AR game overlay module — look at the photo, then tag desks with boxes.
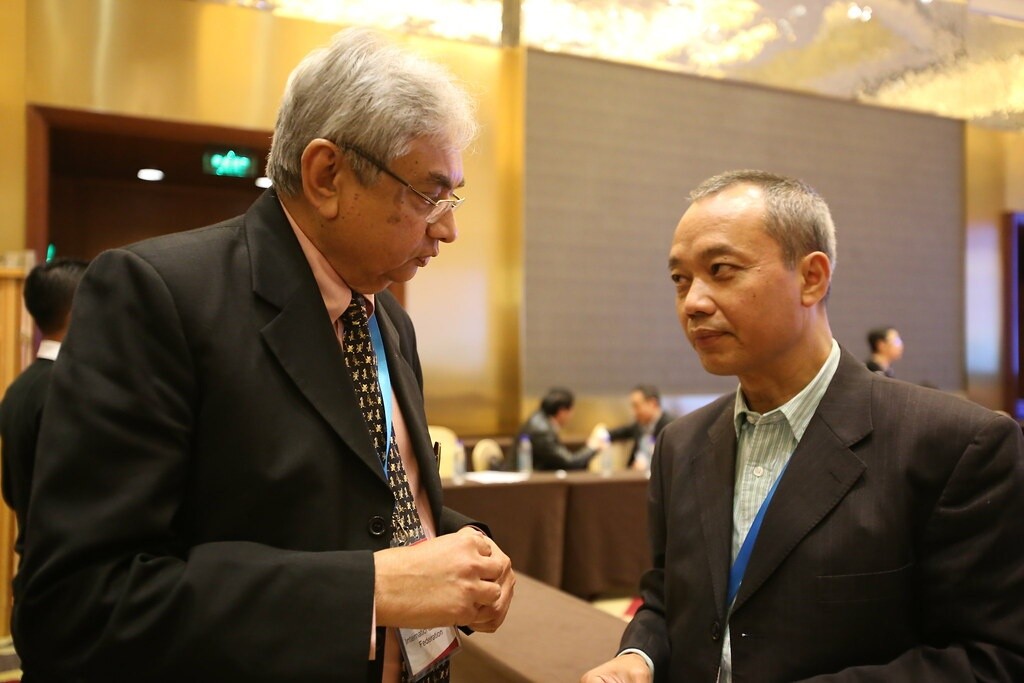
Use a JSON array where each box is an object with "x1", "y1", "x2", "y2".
[{"x1": 439, "y1": 467, "x2": 652, "y2": 683}]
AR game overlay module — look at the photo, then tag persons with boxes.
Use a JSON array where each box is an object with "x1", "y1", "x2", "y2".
[
  {"x1": 0, "y1": 259, "x2": 88, "y2": 683},
  {"x1": 495, "y1": 381, "x2": 675, "y2": 472},
  {"x1": 864, "y1": 325, "x2": 904, "y2": 378},
  {"x1": 7, "y1": 25, "x2": 514, "y2": 683},
  {"x1": 578, "y1": 168, "x2": 1024, "y2": 683}
]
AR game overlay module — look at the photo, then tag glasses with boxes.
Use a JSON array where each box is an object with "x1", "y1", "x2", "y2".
[{"x1": 330, "y1": 140, "x2": 466, "y2": 224}]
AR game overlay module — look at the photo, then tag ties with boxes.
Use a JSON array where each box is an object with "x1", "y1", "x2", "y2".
[{"x1": 335, "y1": 292, "x2": 449, "y2": 683}]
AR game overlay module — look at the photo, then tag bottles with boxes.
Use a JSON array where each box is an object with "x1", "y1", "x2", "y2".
[{"x1": 517, "y1": 433, "x2": 533, "y2": 472}]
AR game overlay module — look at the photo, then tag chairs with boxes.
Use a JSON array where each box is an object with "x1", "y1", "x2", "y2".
[
  {"x1": 588, "y1": 419, "x2": 636, "y2": 471},
  {"x1": 429, "y1": 426, "x2": 458, "y2": 473},
  {"x1": 471, "y1": 439, "x2": 504, "y2": 471}
]
